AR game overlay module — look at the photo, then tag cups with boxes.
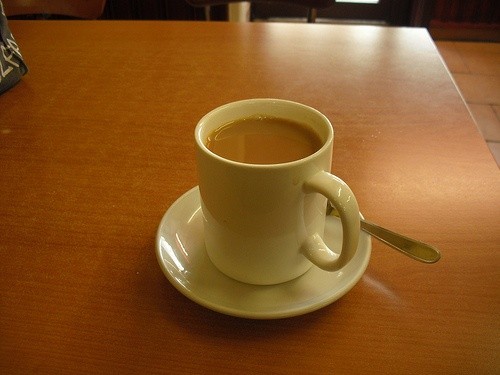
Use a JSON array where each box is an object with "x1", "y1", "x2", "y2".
[{"x1": 194, "y1": 98, "x2": 360, "y2": 286}]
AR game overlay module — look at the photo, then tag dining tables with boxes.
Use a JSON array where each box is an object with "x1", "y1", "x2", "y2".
[{"x1": 0, "y1": 18, "x2": 499, "y2": 375}]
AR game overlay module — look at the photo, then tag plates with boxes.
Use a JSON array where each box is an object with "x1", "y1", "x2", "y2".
[{"x1": 154, "y1": 184, "x2": 371, "y2": 320}]
